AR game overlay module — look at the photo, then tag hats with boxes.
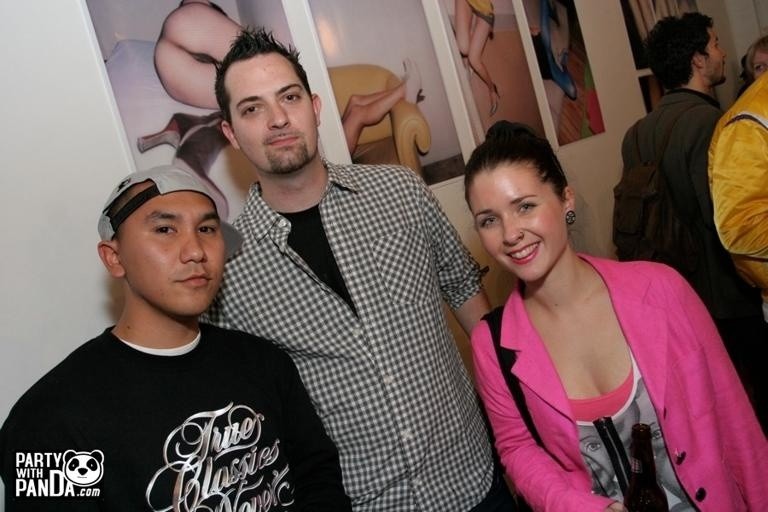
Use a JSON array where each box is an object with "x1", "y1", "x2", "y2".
[{"x1": 97, "y1": 167, "x2": 244, "y2": 262}]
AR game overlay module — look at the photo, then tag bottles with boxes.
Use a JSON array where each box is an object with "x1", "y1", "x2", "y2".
[{"x1": 623, "y1": 422, "x2": 669, "y2": 512}]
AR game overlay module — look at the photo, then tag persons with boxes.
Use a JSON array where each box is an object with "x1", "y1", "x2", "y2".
[
  {"x1": 136, "y1": 1, "x2": 247, "y2": 223},
  {"x1": 1, "y1": 164, "x2": 353, "y2": 512},
  {"x1": 468, "y1": 118, "x2": 767, "y2": 512},
  {"x1": 449, "y1": 0, "x2": 504, "y2": 117},
  {"x1": 191, "y1": 22, "x2": 519, "y2": 512},
  {"x1": 340, "y1": 57, "x2": 422, "y2": 155}
]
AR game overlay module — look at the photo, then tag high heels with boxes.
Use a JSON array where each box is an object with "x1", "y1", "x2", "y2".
[
  {"x1": 490, "y1": 83, "x2": 500, "y2": 116},
  {"x1": 403, "y1": 58, "x2": 426, "y2": 105},
  {"x1": 136, "y1": 112, "x2": 230, "y2": 214}
]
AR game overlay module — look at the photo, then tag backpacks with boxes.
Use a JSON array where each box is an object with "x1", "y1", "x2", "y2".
[{"x1": 612, "y1": 101, "x2": 706, "y2": 274}]
